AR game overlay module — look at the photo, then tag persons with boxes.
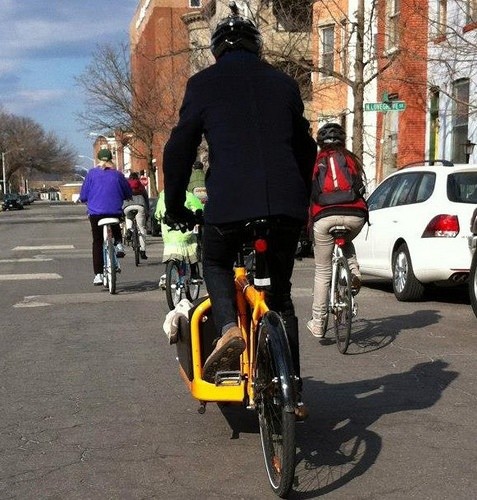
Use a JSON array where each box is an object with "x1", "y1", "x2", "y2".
[
  {"x1": 80, "y1": 149, "x2": 133, "y2": 285},
  {"x1": 307, "y1": 123, "x2": 369, "y2": 338},
  {"x1": 161, "y1": 16, "x2": 318, "y2": 420},
  {"x1": 186, "y1": 161, "x2": 208, "y2": 205},
  {"x1": 154, "y1": 186, "x2": 205, "y2": 288},
  {"x1": 122, "y1": 171, "x2": 150, "y2": 260},
  {"x1": 139, "y1": 170, "x2": 158, "y2": 217}
]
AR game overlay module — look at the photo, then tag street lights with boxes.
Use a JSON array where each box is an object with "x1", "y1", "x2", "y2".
[
  {"x1": 90, "y1": 132, "x2": 119, "y2": 171},
  {"x1": 2, "y1": 148, "x2": 24, "y2": 194},
  {"x1": 74, "y1": 155, "x2": 96, "y2": 181}
]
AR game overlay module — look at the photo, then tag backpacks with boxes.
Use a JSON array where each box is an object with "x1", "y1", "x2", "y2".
[
  {"x1": 312, "y1": 150, "x2": 363, "y2": 207},
  {"x1": 128, "y1": 178, "x2": 141, "y2": 192}
]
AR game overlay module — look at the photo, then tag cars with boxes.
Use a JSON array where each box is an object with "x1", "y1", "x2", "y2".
[
  {"x1": 146, "y1": 197, "x2": 160, "y2": 236},
  {"x1": 23, "y1": 194, "x2": 34, "y2": 205}
]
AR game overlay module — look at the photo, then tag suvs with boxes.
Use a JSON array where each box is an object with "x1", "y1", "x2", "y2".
[
  {"x1": 2, "y1": 193, "x2": 24, "y2": 211},
  {"x1": 336, "y1": 158, "x2": 477, "y2": 317}
]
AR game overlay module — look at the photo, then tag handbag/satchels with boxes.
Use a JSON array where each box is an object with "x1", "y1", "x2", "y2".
[{"x1": 176, "y1": 296, "x2": 218, "y2": 383}]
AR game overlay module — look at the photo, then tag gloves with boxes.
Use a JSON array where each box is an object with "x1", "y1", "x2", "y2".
[{"x1": 163, "y1": 205, "x2": 194, "y2": 233}]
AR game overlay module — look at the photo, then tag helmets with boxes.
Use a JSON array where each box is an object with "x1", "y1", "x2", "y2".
[
  {"x1": 316, "y1": 122, "x2": 345, "y2": 146},
  {"x1": 209, "y1": 16, "x2": 262, "y2": 57}
]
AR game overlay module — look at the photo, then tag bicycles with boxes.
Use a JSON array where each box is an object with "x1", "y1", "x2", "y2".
[
  {"x1": 124, "y1": 209, "x2": 149, "y2": 266},
  {"x1": 161, "y1": 209, "x2": 308, "y2": 498},
  {"x1": 78, "y1": 198, "x2": 132, "y2": 295},
  {"x1": 161, "y1": 246, "x2": 203, "y2": 311},
  {"x1": 312, "y1": 225, "x2": 360, "y2": 355}
]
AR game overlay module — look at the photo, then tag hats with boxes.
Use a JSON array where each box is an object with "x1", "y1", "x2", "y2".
[{"x1": 98, "y1": 149, "x2": 112, "y2": 162}]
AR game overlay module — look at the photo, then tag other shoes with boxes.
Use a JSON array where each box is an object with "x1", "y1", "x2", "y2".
[
  {"x1": 293, "y1": 405, "x2": 307, "y2": 421},
  {"x1": 203, "y1": 327, "x2": 247, "y2": 383},
  {"x1": 115, "y1": 247, "x2": 125, "y2": 257},
  {"x1": 351, "y1": 275, "x2": 360, "y2": 296},
  {"x1": 159, "y1": 274, "x2": 166, "y2": 287},
  {"x1": 191, "y1": 276, "x2": 203, "y2": 285},
  {"x1": 126, "y1": 229, "x2": 132, "y2": 240},
  {"x1": 306, "y1": 320, "x2": 323, "y2": 338},
  {"x1": 141, "y1": 251, "x2": 148, "y2": 259},
  {"x1": 93, "y1": 276, "x2": 102, "y2": 285}
]
